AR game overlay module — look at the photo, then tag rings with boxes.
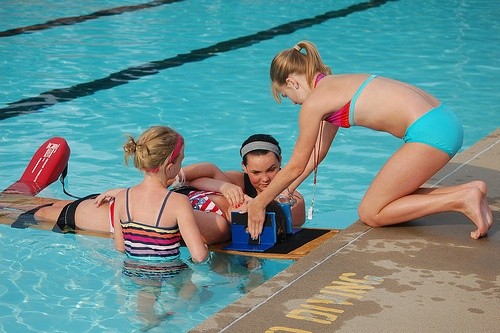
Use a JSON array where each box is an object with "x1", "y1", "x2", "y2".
[{"x1": 225, "y1": 195, "x2": 230, "y2": 197}]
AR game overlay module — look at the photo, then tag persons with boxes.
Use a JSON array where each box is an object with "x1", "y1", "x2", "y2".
[
  {"x1": 182, "y1": 134, "x2": 306, "y2": 293},
  {"x1": 1, "y1": 163, "x2": 287, "y2": 245},
  {"x1": 241, "y1": 41, "x2": 494, "y2": 240},
  {"x1": 113, "y1": 125, "x2": 208, "y2": 332}
]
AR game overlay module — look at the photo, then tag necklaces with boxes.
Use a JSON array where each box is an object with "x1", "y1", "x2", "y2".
[{"x1": 307, "y1": 73, "x2": 329, "y2": 219}]
